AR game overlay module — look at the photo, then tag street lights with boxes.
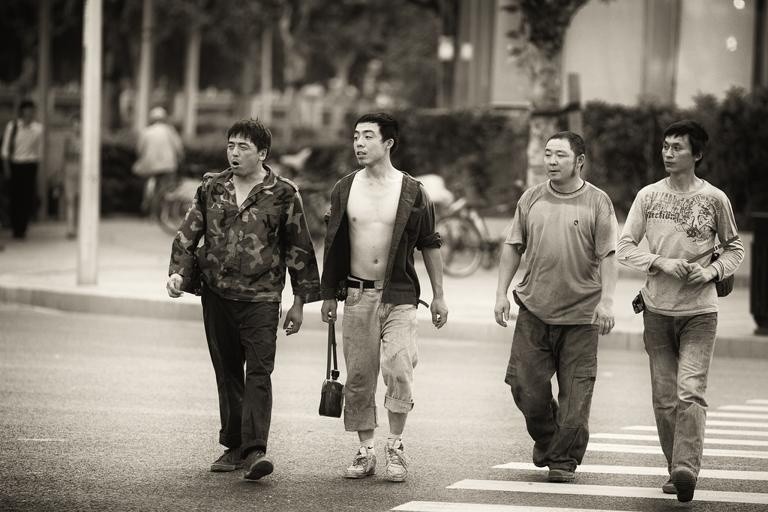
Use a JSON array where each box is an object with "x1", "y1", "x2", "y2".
[{"x1": 436, "y1": 32, "x2": 457, "y2": 111}]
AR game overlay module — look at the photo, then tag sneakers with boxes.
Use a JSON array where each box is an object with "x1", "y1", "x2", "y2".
[
  {"x1": 548, "y1": 469, "x2": 575, "y2": 482},
  {"x1": 532, "y1": 442, "x2": 546, "y2": 467},
  {"x1": 384, "y1": 439, "x2": 408, "y2": 481},
  {"x1": 662, "y1": 478, "x2": 676, "y2": 494},
  {"x1": 243, "y1": 450, "x2": 274, "y2": 480},
  {"x1": 343, "y1": 446, "x2": 377, "y2": 479},
  {"x1": 671, "y1": 467, "x2": 696, "y2": 502},
  {"x1": 210, "y1": 448, "x2": 242, "y2": 472}
]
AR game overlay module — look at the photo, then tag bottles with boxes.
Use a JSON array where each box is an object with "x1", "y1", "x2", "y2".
[{"x1": 319, "y1": 368, "x2": 345, "y2": 418}]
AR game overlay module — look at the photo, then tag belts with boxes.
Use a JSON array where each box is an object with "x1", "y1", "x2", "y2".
[{"x1": 345, "y1": 281, "x2": 373, "y2": 288}]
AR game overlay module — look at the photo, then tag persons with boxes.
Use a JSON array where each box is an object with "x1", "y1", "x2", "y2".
[
  {"x1": 55, "y1": 114, "x2": 80, "y2": 238},
  {"x1": 132, "y1": 107, "x2": 186, "y2": 216},
  {"x1": 321, "y1": 113, "x2": 448, "y2": 482},
  {"x1": 495, "y1": 131, "x2": 621, "y2": 482},
  {"x1": 1, "y1": 98, "x2": 44, "y2": 239},
  {"x1": 616, "y1": 119, "x2": 745, "y2": 502},
  {"x1": 166, "y1": 117, "x2": 321, "y2": 480}
]
